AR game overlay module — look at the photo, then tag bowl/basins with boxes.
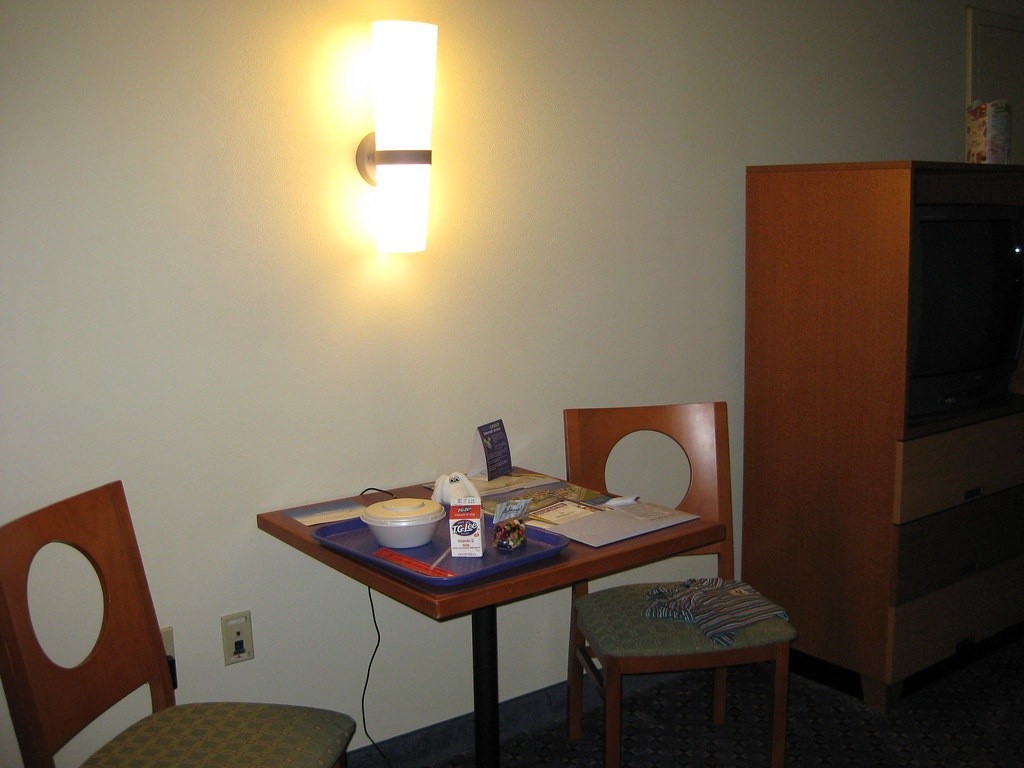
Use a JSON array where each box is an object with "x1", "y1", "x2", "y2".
[{"x1": 360, "y1": 498, "x2": 446, "y2": 549}]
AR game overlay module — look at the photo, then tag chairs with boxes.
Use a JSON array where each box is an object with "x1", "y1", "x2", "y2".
[
  {"x1": 0, "y1": 481, "x2": 356, "y2": 768},
  {"x1": 563, "y1": 402, "x2": 797, "y2": 768}
]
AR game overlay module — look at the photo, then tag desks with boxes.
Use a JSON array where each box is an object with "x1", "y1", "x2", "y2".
[{"x1": 257, "y1": 466, "x2": 726, "y2": 768}]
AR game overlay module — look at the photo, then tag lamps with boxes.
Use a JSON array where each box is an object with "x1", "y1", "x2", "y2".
[{"x1": 356, "y1": 21, "x2": 438, "y2": 252}]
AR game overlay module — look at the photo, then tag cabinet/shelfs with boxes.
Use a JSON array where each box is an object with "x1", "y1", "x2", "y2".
[{"x1": 740, "y1": 160, "x2": 1024, "y2": 712}]
